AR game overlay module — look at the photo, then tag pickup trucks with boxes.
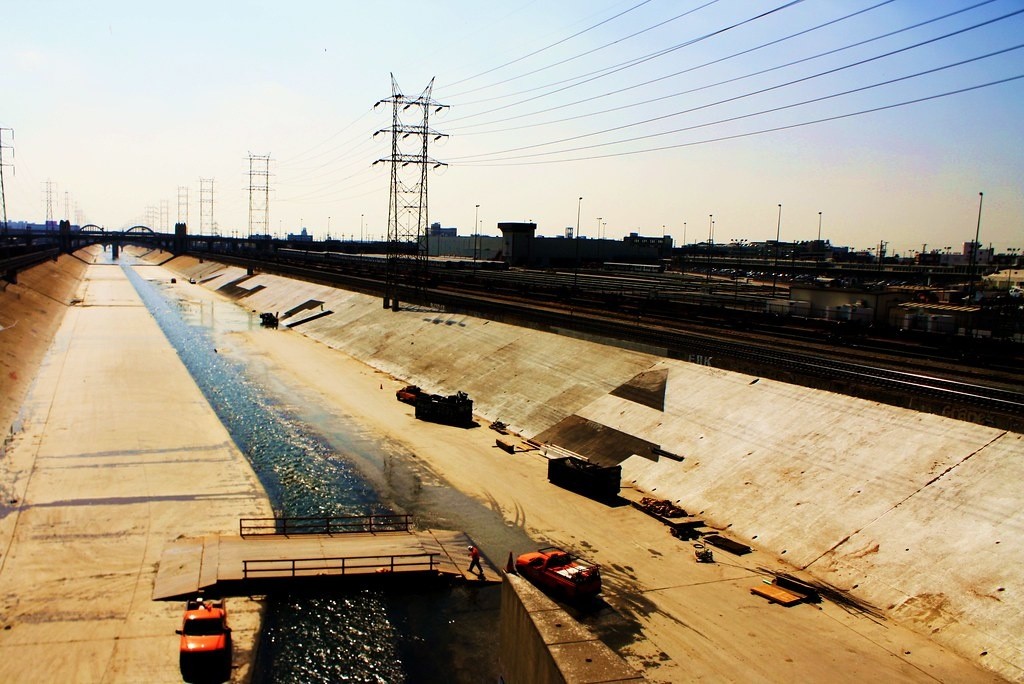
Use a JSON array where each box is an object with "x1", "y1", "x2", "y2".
[
  {"x1": 516, "y1": 550, "x2": 605, "y2": 607},
  {"x1": 175, "y1": 599, "x2": 233, "y2": 666}
]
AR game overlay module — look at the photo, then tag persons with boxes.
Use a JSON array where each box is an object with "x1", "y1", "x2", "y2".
[{"x1": 467, "y1": 546, "x2": 484, "y2": 576}]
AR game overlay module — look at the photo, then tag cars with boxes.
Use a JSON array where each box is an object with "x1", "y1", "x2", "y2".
[{"x1": 396, "y1": 384, "x2": 426, "y2": 403}]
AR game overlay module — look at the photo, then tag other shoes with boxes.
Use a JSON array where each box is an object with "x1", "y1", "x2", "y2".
[
  {"x1": 467, "y1": 569, "x2": 472, "y2": 572},
  {"x1": 480, "y1": 570, "x2": 483, "y2": 573}
]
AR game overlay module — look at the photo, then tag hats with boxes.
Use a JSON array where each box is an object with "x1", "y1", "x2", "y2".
[{"x1": 468, "y1": 546, "x2": 473, "y2": 550}]
